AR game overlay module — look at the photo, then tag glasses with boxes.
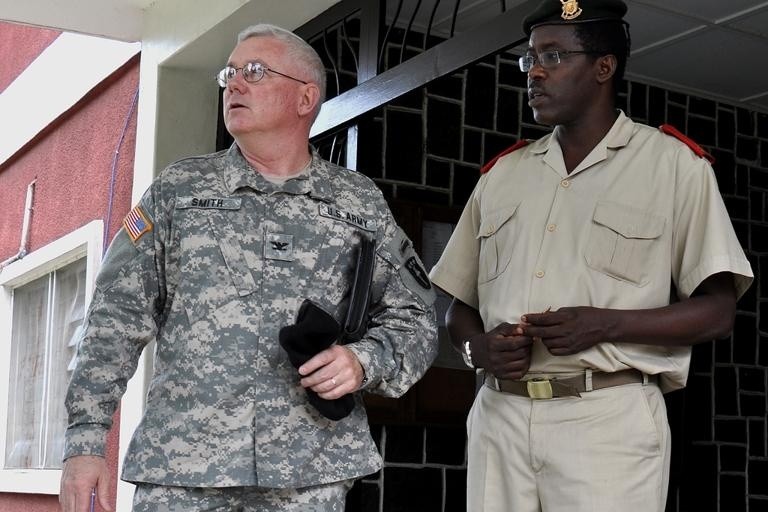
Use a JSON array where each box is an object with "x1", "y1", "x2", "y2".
[
  {"x1": 214, "y1": 62, "x2": 308, "y2": 88},
  {"x1": 518, "y1": 50, "x2": 586, "y2": 73}
]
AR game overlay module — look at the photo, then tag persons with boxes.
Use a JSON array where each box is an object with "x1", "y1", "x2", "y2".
[
  {"x1": 423, "y1": 0, "x2": 757, "y2": 511},
  {"x1": 55, "y1": 20, "x2": 441, "y2": 511}
]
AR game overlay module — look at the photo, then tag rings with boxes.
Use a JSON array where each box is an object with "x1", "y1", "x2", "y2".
[{"x1": 330, "y1": 377, "x2": 337, "y2": 386}]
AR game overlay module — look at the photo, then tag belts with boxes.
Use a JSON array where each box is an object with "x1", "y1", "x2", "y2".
[{"x1": 483, "y1": 368, "x2": 655, "y2": 400}]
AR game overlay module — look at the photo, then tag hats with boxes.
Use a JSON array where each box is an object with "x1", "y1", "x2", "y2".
[
  {"x1": 278, "y1": 301, "x2": 356, "y2": 421},
  {"x1": 521, "y1": 0, "x2": 627, "y2": 36}
]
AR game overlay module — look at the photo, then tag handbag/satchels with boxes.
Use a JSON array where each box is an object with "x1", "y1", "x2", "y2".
[{"x1": 339, "y1": 238, "x2": 376, "y2": 346}]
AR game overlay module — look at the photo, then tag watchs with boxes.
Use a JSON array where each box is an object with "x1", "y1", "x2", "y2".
[{"x1": 460, "y1": 338, "x2": 476, "y2": 370}]
